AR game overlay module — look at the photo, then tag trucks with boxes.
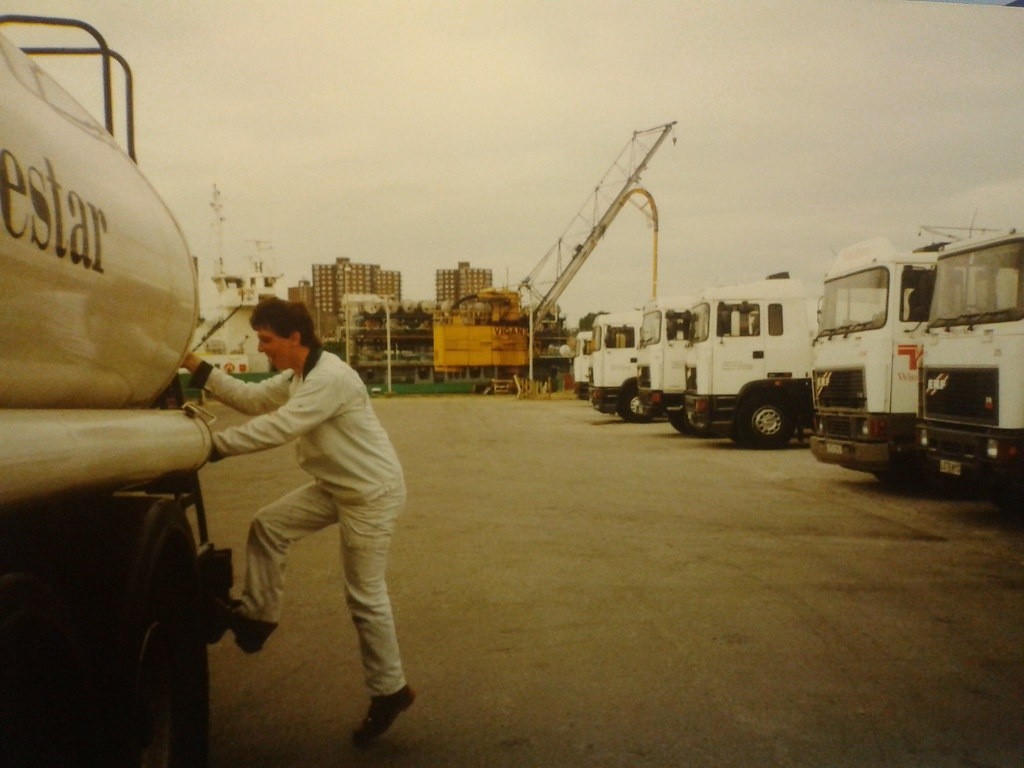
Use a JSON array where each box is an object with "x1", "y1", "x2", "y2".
[
  {"x1": 573, "y1": 332, "x2": 626, "y2": 400},
  {"x1": 810, "y1": 234, "x2": 1017, "y2": 499},
  {"x1": 587, "y1": 310, "x2": 683, "y2": 423},
  {"x1": 683, "y1": 271, "x2": 913, "y2": 451},
  {"x1": 919, "y1": 229, "x2": 1024, "y2": 521},
  {"x1": 0, "y1": 15, "x2": 233, "y2": 768},
  {"x1": 636, "y1": 299, "x2": 759, "y2": 437}
]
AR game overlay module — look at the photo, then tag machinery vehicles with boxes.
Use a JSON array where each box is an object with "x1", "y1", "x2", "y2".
[{"x1": 431, "y1": 121, "x2": 678, "y2": 374}]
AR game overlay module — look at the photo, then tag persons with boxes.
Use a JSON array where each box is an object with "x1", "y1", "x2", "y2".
[{"x1": 183, "y1": 295, "x2": 414, "y2": 747}]
